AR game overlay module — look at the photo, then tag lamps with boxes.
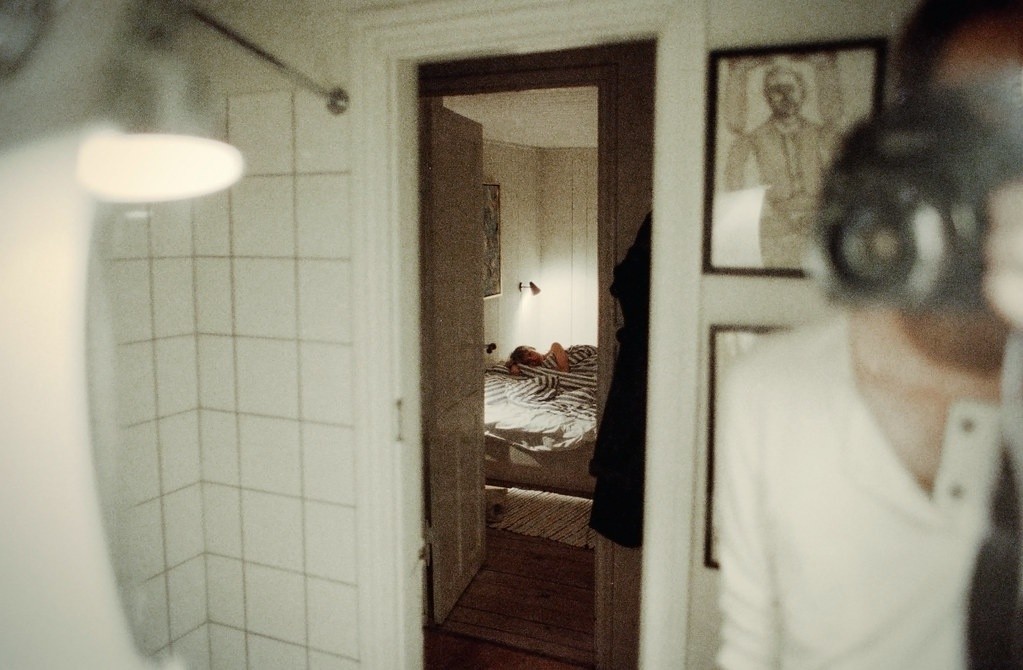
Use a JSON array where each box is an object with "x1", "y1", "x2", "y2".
[
  {"x1": 81, "y1": 1, "x2": 350, "y2": 206},
  {"x1": 519, "y1": 281, "x2": 541, "y2": 296}
]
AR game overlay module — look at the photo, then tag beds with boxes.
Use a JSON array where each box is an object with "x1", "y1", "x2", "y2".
[{"x1": 482, "y1": 360, "x2": 595, "y2": 549}]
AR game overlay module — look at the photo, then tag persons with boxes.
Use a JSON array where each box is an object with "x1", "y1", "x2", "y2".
[
  {"x1": 715, "y1": 0, "x2": 1023, "y2": 670},
  {"x1": 505, "y1": 342, "x2": 569, "y2": 375}
]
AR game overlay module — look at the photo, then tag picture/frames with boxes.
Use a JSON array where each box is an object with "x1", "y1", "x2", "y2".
[
  {"x1": 702, "y1": 36, "x2": 886, "y2": 279},
  {"x1": 705, "y1": 323, "x2": 789, "y2": 570},
  {"x1": 482, "y1": 183, "x2": 503, "y2": 300}
]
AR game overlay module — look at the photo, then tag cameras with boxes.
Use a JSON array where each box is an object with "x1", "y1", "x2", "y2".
[{"x1": 810, "y1": 83, "x2": 1023, "y2": 316}]
